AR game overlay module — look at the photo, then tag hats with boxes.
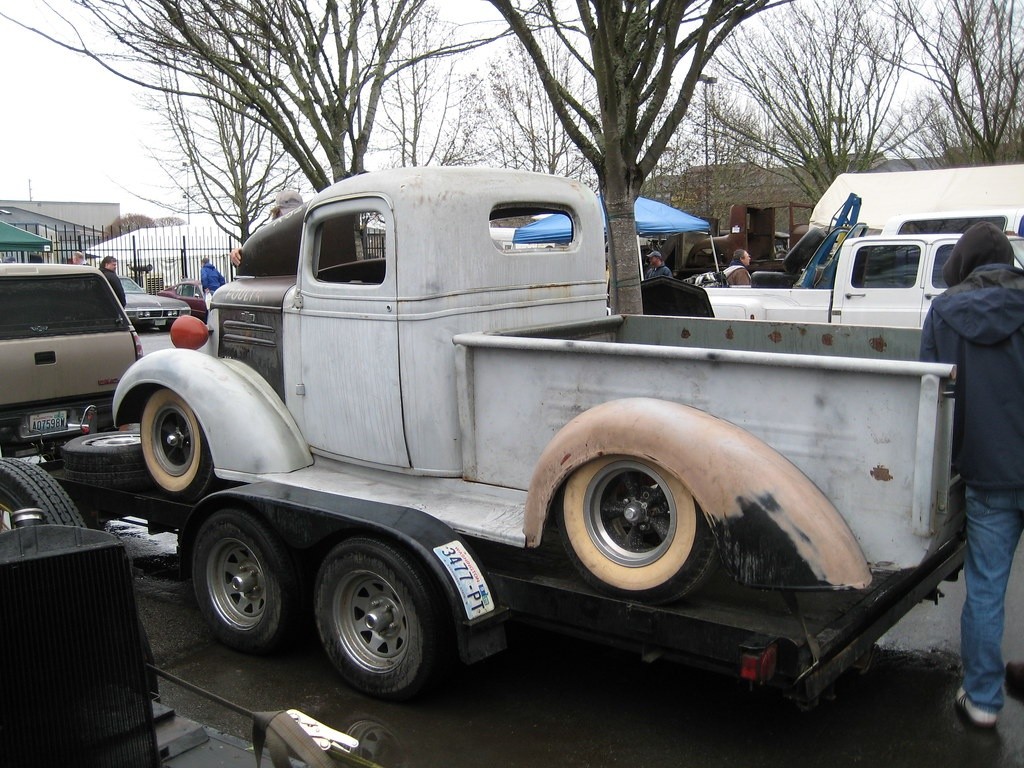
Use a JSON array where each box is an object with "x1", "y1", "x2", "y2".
[{"x1": 646, "y1": 252, "x2": 662, "y2": 258}]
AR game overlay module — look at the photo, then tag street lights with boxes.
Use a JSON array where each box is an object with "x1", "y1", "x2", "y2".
[{"x1": 697, "y1": 74, "x2": 717, "y2": 216}]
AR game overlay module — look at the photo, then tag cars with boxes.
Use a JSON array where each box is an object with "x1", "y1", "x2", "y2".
[
  {"x1": 116, "y1": 275, "x2": 191, "y2": 332},
  {"x1": 157, "y1": 279, "x2": 208, "y2": 324},
  {"x1": 0, "y1": 263, "x2": 143, "y2": 456}
]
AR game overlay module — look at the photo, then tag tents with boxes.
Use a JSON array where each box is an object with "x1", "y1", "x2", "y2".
[
  {"x1": 512, "y1": 195, "x2": 719, "y2": 282},
  {"x1": 0, "y1": 220, "x2": 53, "y2": 264}
]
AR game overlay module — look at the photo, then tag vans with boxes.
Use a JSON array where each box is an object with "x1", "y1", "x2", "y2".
[{"x1": 808, "y1": 163, "x2": 1023, "y2": 237}]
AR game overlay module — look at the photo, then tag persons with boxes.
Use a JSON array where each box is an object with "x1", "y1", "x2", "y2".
[
  {"x1": 98, "y1": 256, "x2": 128, "y2": 309},
  {"x1": 73, "y1": 252, "x2": 90, "y2": 266},
  {"x1": 645, "y1": 251, "x2": 672, "y2": 280},
  {"x1": 230, "y1": 190, "x2": 304, "y2": 268},
  {"x1": 723, "y1": 250, "x2": 751, "y2": 285},
  {"x1": 919, "y1": 222, "x2": 1024, "y2": 724},
  {"x1": 3, "y1": 253, "x2": 44, "y2": 263},
  {"x1": 201, "y1": 258, "x2": 225, "y2": 310}
]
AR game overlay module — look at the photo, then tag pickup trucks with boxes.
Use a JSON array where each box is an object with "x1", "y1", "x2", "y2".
[
  {"x1": 702, "y1": 233, "x2": 1024, "y2": 329},
  {"x1": 111, "y1": 167, "x2": 958, "y2": 609}
]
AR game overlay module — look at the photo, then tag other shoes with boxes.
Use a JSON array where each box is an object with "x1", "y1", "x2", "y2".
[{"x1": 957, "y1": 686, "x2": 997, "y2": 727}]
